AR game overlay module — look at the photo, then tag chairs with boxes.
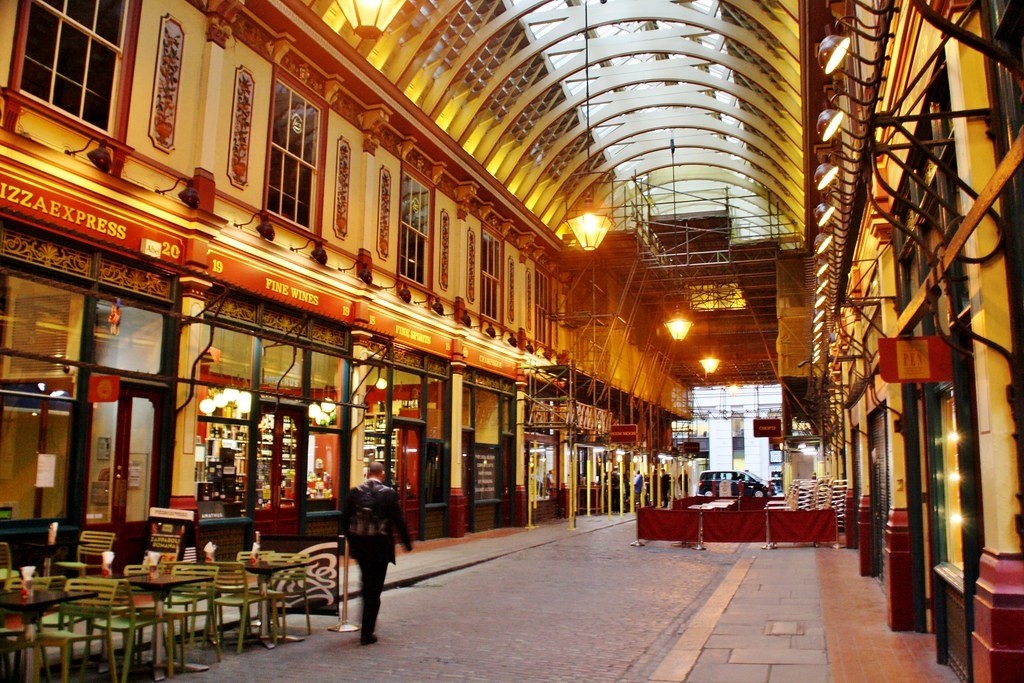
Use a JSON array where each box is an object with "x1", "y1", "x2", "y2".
[{"x1": 0, "y1": 531, "x2": 311, "y2": 683}]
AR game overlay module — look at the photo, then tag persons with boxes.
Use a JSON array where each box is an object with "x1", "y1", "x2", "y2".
[
  {"x1": 546, "y1": 470, "x2": 554, "y2": 488},
  {"x1": 343, "y1": 460, "x2": 413, "y2": 645},
  {"x1": 611, "y1": 465, "x2": 688, "y2": 513}
]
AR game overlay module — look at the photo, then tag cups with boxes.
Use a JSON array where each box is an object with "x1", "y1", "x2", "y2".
[
  {"x1": 250, "y1": 552, "x2": 259, "y2": 565},
  {"x1": 22, "y1": 580, "x2": 33, "y2": 598},
  {"x1": 205, "y1": 552, "x2": 215, "y2": 561},
  {"x1": 149, "y1": 565, "x2": 158, "y2": 578},
  {"x1": 102, "y1": 564, "x2": 112, "y2": 578}
]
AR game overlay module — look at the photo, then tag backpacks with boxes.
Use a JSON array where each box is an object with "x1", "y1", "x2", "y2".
[{"x1": 349, "y1": 485, "x2": 390, "y2": 535}]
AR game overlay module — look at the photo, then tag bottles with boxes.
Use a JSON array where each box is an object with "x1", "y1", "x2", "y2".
[{"x1": 364, "y1": 416, "x2": 395, "y2": 480}]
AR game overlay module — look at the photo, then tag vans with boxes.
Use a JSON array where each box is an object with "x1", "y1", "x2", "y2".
[{"x1": 698, "y1": 471, "x2": 777, "y2": 498}]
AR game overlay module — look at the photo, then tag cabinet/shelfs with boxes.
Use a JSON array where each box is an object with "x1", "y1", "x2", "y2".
[
  {"x1": 364, "y1": 413, "x2": 398, "y2": 479},
  {"x1": 205, "y1": 413, "x2": 296, "y2": 513}
]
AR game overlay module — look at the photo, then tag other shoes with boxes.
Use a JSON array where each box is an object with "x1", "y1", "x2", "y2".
[{"x1": 361, "y1": 635, "x2": 376, "y2": 645}]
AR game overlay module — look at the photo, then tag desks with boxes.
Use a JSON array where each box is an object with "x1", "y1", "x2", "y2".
[
  {"x1": 12, "y1": 538, "x2": 90, "y2": 577},
  {"x1": 111, "y1": 573, "x2": 214, "y2": 682},
  {"x1": 763, "y1": 501, "x2": 790, "y2": 511},
  {"x1": 223, "y1": 560, "x2": 312, "y2": 649},
  {"x1": 0, "y1": 588, "x2": 99, "y2": 683},
  {"x1": 688, "y1": 499, "x2": 736, "y2": 511}
]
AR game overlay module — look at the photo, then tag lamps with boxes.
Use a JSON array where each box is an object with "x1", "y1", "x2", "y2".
[
  {"x1": 664, "y1": 140, "x2": 694, "y2": 341},
  {"x1": 338, "y1": 260, "x2": 372, "y2": 286},
  {"x1": 335, "y1": 0, "x2": 406, "y2": 42},
  {"x1": 379, "y1": 280, "x2": 411, "y2": 303},
  {"x1": 234, "y1": 209, "x2": 275, "y2": 242},
  {"x1": 65, "y1": 137, "x2": 111, "y2": 173},
  {"x1": 818, "y1": 16, "x2": 880, "y2": 75},
  {"x1": 817, "y1": 93, "x2": 847, "y2": 142},
  {"x1": 810, "y1": 149, "x2": 857, "y2": 365},
  {"x1": 565, "y1": 0, "x2": 613, "y2": 250},
  {"x1": 698, "y1": 311, "x2": 721, "y2": 373},
  {"x1": 155, "y1": 178, "x2": 200, "y2": 210},
  {"x1": 290, "y1": 238, "x2": 328, "y2": 265},
  {"x1": 413, "y1": 294, "x2": 551, "y2": 362}
]
